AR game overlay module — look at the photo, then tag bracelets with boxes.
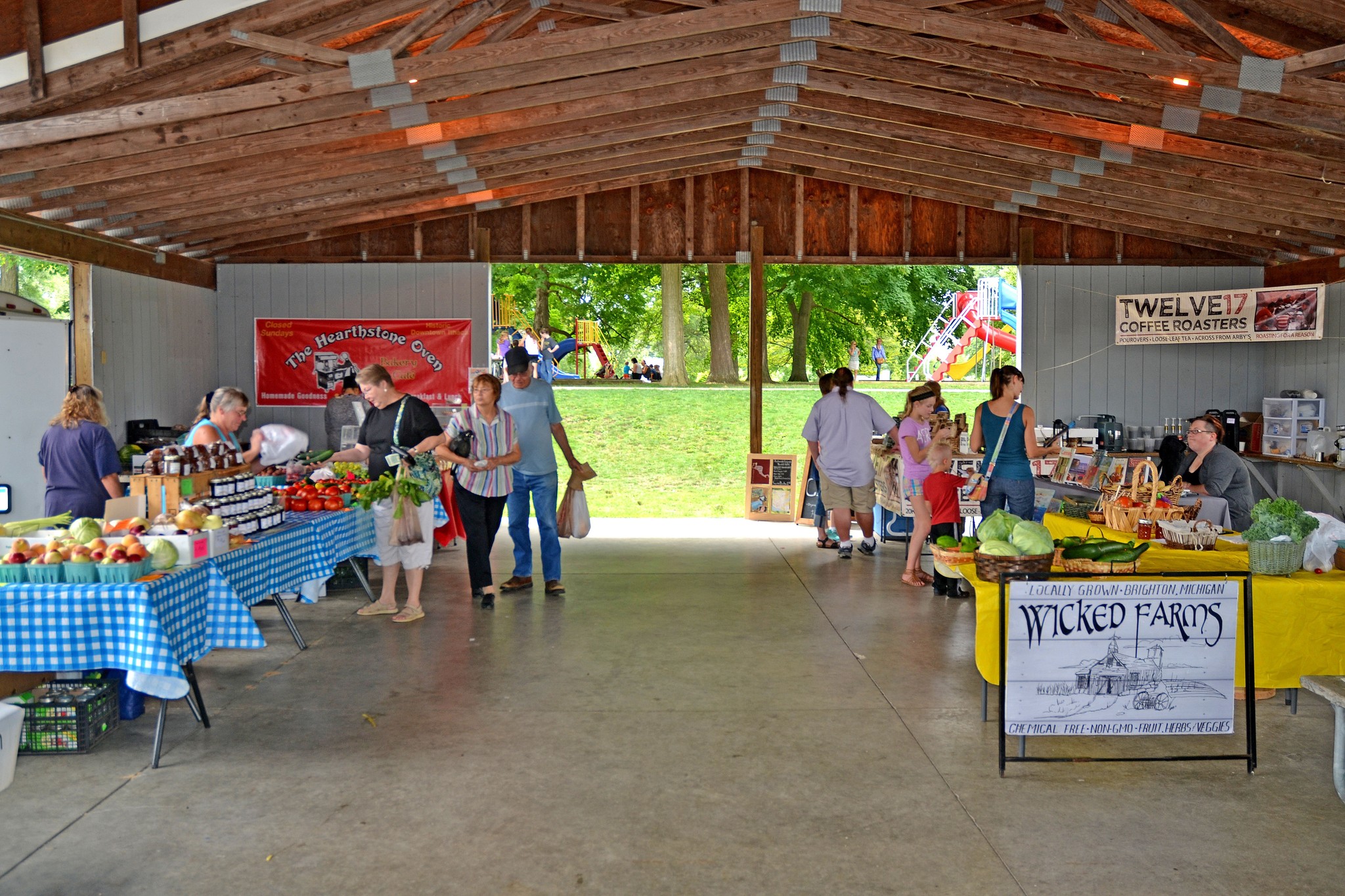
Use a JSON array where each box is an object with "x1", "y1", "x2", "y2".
[{"x1": 412, "y1": 447, "x2": 419, "y2": 454}]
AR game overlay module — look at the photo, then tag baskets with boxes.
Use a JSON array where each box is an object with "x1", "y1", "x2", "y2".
[
  {"x1": 12, "y1": 676, "x2": 122, "y2": 755},
  {"x1": 946, "y1": 423, "x2": 969, "y2": 452},
  {"x1": 929, "y1": 460, "x2": 1306, "y2": 584}
]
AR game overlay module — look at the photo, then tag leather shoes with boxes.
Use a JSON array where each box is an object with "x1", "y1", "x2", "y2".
[
  {"x1": 472, "y1": 586, "x2": 495, "y2": 610},
  {"x1": 500, "y1": 576, "x2": 533, "y2": 591},
  {"x1": 544, "y1": 580, "x2": 566, "y2": 596}
]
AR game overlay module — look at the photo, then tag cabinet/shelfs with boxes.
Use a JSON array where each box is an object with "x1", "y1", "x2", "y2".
[{"x1": 1261, "y1": 397, "x2": 1326, "y2": 459}]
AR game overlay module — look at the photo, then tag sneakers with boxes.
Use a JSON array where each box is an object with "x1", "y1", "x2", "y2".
[
  {"x1": 857, "y1": 536, "x2": 877, "y2": 556},
  {"x1": 838, "y1": 544, "x2": 853, "y2": 559}
]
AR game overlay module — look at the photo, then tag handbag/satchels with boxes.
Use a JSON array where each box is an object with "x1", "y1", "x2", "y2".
[
  {"x1": 449, "y1": 430, "x2": 475, "y2": 458},
  {"x1": 388, "y1": 462, "x2": 423, "y2": 545},
  {"x1": 397, "y1": 447, "x2": 442, "y2": 504},
  {"x1": 556, "y1": 486, "x2": 591, "y2": 539},
  {"x1": 258, "y1": 420, "x2": 309, "y2": 467},
  {"x1": 877, "y1": 358, "x2": 884, "y2": 364}
]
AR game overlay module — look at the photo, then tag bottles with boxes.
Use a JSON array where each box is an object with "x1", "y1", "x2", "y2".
[{"x1": 1163, "y1": 425, "x2": 1184, "y2": 443}]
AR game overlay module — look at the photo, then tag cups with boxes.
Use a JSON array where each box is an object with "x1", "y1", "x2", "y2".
[
  {"x1": 1302, "y1": 389, "x2": 1318, "y2": 398},
  {"x1": 1137, "y1": 518, "x2": 1152, "y2": 540},
  {"x1": 1155, "y1": 520, "x2": 1169, "y2": 539},
  {"x1": 1315, "y1": 451, "x2": 1325, "y2": 462},
  {"x1": 1239, "y1": 441, "x2": 1246, "y2": 452},
  {"x1": 1121, "y1": 422, "x2": 1164, "y2": 452}
]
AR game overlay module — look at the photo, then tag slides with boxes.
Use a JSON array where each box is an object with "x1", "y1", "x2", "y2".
[
  {"x1": 536, "y1": 352, "x2": 580, "y2": 379},
  {"x1": 960, "y1": 295, "x2": 1016, "y2": 355},
  {"x1": 550, "y1": 338, "x2": 579, "y2": 367},
  {"x1": 1001, "y1": 310, "x2": 1016, "y2": 330},
  {"x1": 946, "y1": 323, "x2": 1013, "y2": 379}
]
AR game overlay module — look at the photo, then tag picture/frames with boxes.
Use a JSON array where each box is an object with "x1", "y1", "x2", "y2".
[
  {"x1": 745, "y1": 452, "x2": 796, "y2": 524},
  {"x1": 794, "y1": 440, "x2": 831, "y2": 528}
]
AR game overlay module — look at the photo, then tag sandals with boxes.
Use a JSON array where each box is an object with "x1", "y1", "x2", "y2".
[
  {"x1": 915, "y1": 566, "x2": 935, "y2": 584},
  {"x1": 392, "y1": 603, "x2": 424, "y2": 622},
  {"x1": 901, "y1": 569, "x2": 925, "y2": 587},
  {"x1": 816, "y1": 535, "x2": 839, "y2": 549},
  {"x1": 357, "y1": 600, "x2": 399, "y2": 614}
]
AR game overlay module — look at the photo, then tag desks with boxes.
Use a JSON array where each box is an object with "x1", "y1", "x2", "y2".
[
  {"x1": 1239, "y1": 450, "x2": 1344, "y2": 521},
  {"x1": 930, "y1": 512, "x2": 1345, "y2": 765},
  {"x1": 1, "y1": 480, "x2": 451, "y2": 771},
  {"x1": 870, "y1": 440, "x2": 1161, "y2": 559}
]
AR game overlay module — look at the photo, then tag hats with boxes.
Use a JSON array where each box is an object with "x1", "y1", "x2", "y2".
[
  {"x1": 505, "y1": 346, "x2": 531, "y2": 375},
  {"x1": 342, "y1": 375, "x2": 360, "y2": 389}
]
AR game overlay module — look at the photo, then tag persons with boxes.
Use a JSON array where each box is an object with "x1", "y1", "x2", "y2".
[
  {"x1": 37, "y1": 384, "x2": 124, "y2": 529},
  {"x1": 512, "y1": 339, "x2": 519, "y2": 347},
  {"x1": 898, "y1": 386, "x2": 951, "y2": 587},
  {"x1": 871, "y1": 338, "x2": 886, "y2": 381},
  {"x1": 497, "y1": 346, "x2": 582, "y2": 595},
  {"x1": 623, "y1": 361, "x2": 631, "y2": 379},
  {"x1": 1254, "y1": 292, "x2": 1314, "y2": 330},
  {"x1": 433, "y1": 373, "x2": 522, "y2": 609},
  {"x1": 496, "y1": 331, "x2": 512, "y2": 384},
  {"x1": 497, "y1": 334, "x2": 512, "y2": 380},
  {"x1": 641, "y1": 360, "x2": 651, "y2": 380},
  {"x1": 519, "y1": 328, "x2": 539, "y2": 379},
  {"x1": 812, "y1": 373, "x2": 841, "y2": 549},
  {"x1": 630, "y1": 358, "x2": 642, "y2": 379},
  {"x1": 848, "y1": 341, "x2": 861, "y2": 382},
  {"x1": 922, "y1": 440, "x2": 974, "y2": 598},
  {"x1": 184, "y1": 386, "x2": 268, "y2": 475},
  {"x1": 923, "y1": 380, "x2": 950, "y2": 414},
  {"x1": 649, "y1": 365, "x2": 657, "y2": 379},
  {"x1": 1168, "y1": 414, "x2": 1256, "y2": 532},
  {"x1": 969, "y1": 365, "x2": 1060, "y2": 521},
  {"x1": 324, "y1": 375, "x2": 373, "y2": 452},
  {"x1": 303, "y1": 364, "x2": 446, "y2": 623},
  {"x1": 539, "y1": 328, "x2": 559, "y2": 385},
  {"x1": 800, "y1": 367, "x2": 901, "y2": 559}
]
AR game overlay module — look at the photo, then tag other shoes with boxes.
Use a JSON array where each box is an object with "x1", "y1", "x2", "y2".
[
  {"x1": 934, "y1": 588, "x2": 948, "y2": 596},
  {"x1": 949, "y1": 591, "x2": 970, "y2": 598}
]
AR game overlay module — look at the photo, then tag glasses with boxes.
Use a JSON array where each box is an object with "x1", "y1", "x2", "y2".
[
  {"x1": 360, "y1": 385, "x2": 376, "y2": 396},
  {"x1": 1185, "y1": 429, "x2": 1212, "y2": 434},
  {"x1": 471, "y1": 388, "x2": 493, "y2": 394},
  {"x1": 854, "y1": 344, "x2": 856, "y2": 345},
  {"x1": 234, "y1": 410, "x2": 249, "y2": 417},
  {"x1": 878, "y1": 341, "x2": 882, "y2": 342}
]
